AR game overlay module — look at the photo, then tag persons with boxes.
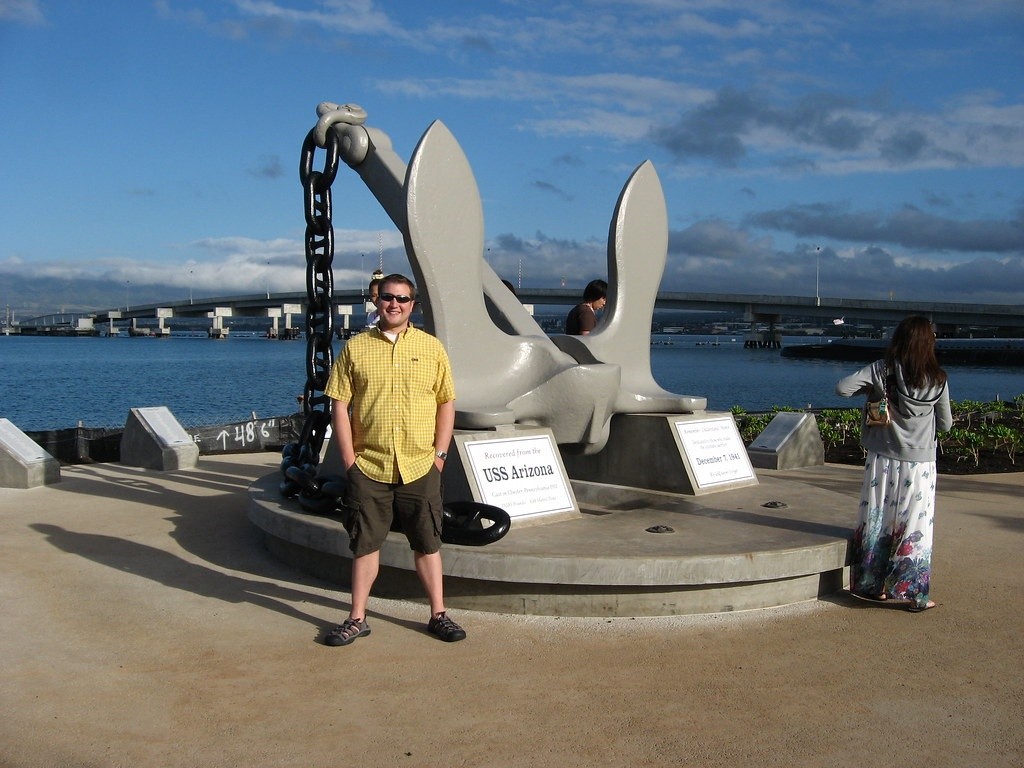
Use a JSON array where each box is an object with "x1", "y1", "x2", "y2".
[
  {"x1": 565, "y1": 278, "x2": 610, "y2": 336},
  {"x1": 359, "y1": 269, "x2": 385, "y2": 333},
  {"x1": 323, "y1": 273, "x2": 467, "y2": 647},
  {"x1": 833, "y1": 313, "x2": 954, "y2": 610}
]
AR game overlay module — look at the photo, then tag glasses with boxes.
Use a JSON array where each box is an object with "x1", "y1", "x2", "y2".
[{"x1": 378, "y1": 293, "x2": 412, "y2": 303}]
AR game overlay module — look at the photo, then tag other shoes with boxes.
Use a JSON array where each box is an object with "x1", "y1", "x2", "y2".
[
  {"x1": 909, "y1": 599, "x2": 936, "y2": 612},
  {"x1": 851, "y1": 589, "x2": 888, "y2": 603}
]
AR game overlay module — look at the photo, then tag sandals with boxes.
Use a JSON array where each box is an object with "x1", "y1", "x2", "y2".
[
  {"x1": 427, "y1": 610, "x2": 466, "y2": 641},
  {"x1": 325, "y1": 615, "x2": 371, "y2": 646}
]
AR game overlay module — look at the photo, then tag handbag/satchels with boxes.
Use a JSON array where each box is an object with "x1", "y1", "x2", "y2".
[{"x1": 865, "y1": 358, "x2": 890, "y2": 425}]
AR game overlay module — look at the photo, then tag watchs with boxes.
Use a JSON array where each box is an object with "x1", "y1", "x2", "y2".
[{"x1": 434, "y1": 450, "x2": 448, "y2": 462}]
